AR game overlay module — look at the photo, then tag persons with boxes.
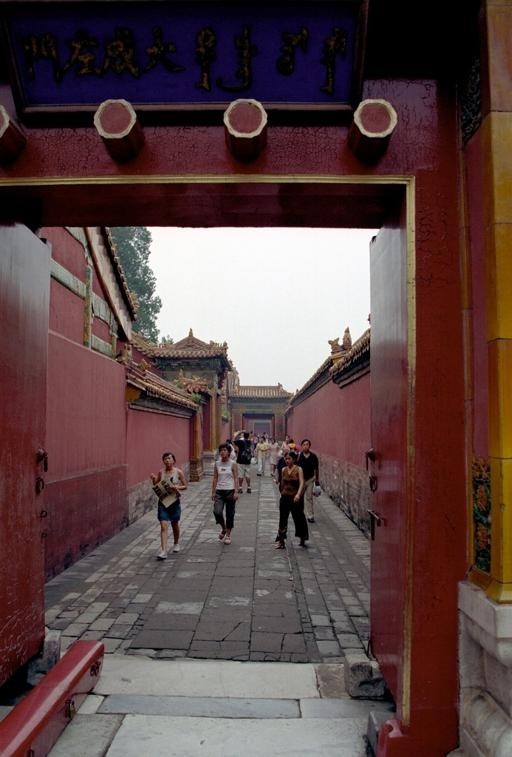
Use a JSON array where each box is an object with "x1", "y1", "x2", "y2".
[
  {"x1": 211, "y1": 429, "x2": 320, "y2": 549},
  {"x1": 150, "y1": 451, "x2": 189, "y2": 560}
]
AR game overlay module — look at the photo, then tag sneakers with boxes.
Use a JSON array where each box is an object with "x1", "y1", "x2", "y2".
[
  {"x1": 157, "y1": 550, "x2": 167, "y2": 559},
  {"x1": 218, "y1": 530, "x2": 231, "y2": 545},
  {"x1": 172, "y1": 544, "x2": 181, "y2": 552}
]
